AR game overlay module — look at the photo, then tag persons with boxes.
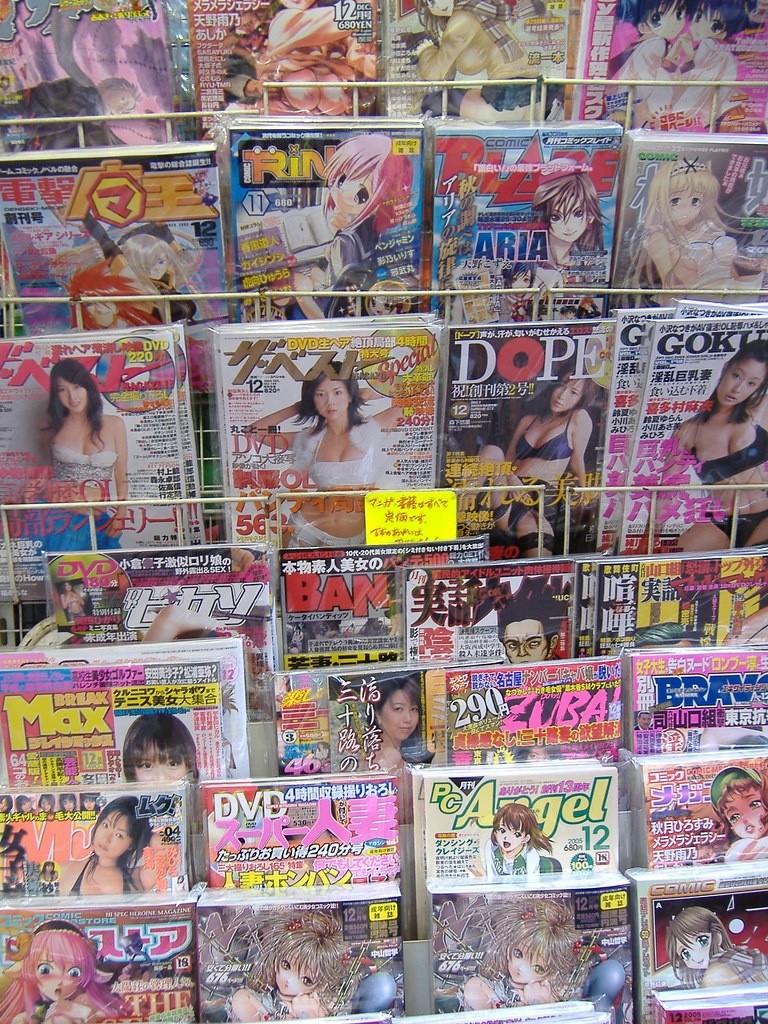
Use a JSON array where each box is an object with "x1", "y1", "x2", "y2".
[
  {"x1": 455, "y1": 368, "x2": 598, "y2": 557},
  {"x1": 238, "y1": 360, "x2": 410, "y2": 551},
  {"x1": 120, "y1": 709, "x2": 196, "y2": 783},
  {"x1": 0, "y1": 789, "x2": 157, "y2": 898},
  {"x1": 22, "y1": 359, "x2": 129, "y2": 551},
  {"x1": 650, "y1": 339, "x2": 768, "y2": 555},
  {"x1": 363, "y1": 675, "x2": 421, "y2": 774}
]
